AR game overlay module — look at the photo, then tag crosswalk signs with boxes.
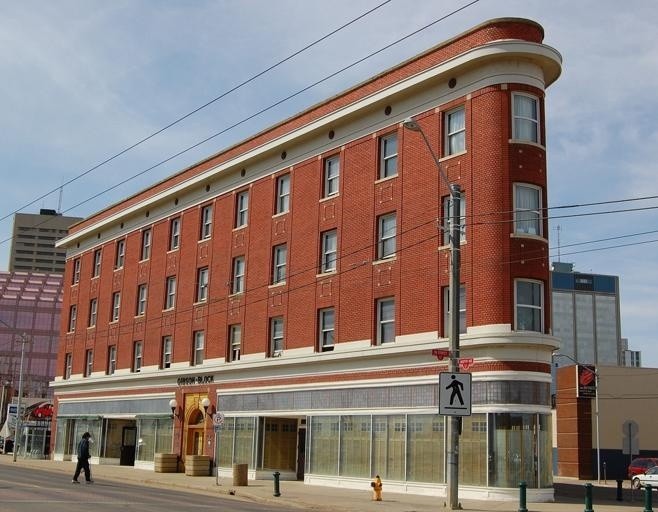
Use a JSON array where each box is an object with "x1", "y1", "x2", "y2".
[{"x1": 438, "y1": 372, "x2": 471, "y2": 416}]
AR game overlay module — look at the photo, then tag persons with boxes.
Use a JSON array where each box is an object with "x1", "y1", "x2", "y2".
[{"x1": 72, "y1": 432, "x2": 95, "y2": 484}]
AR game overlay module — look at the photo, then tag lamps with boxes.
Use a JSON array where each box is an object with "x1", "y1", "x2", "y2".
[
  {"x1": 168, "y1": 399, "x2": 179, "y2": 420},
  {"x1": 201, "y1": 398, "x2": 212, "y2": 418}
]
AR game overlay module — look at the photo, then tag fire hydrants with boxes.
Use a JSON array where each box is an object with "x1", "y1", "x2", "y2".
[{"x1": 370, "y1": 474, "x2": 383, "y2": 501}]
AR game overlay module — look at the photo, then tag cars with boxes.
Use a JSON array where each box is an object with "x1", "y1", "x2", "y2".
[
  {"x1": 31, "y1": 401, "x2": 53, "y2": 420},
  {"x1": 632, "y1": 465, "x2": 657, "y2": 489},
  {"x1": 0, "y1": 435, "x2": 14, "y2": 455},
  {"x1": 627, "y1": 457, "x2": 657, "y2": 481}
]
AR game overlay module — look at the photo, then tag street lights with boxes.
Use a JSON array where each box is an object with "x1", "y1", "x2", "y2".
[
  {"x1": 0, "y1": 318, "x2": 30, "y2": 463},
  {"x1": 552, "y1": 353, "x2": 601, "y2": 484},
  {"x1": 403, "y1": 117, "x2": 460, "y2": 509}
]
